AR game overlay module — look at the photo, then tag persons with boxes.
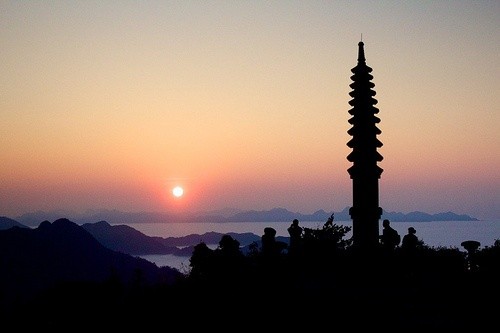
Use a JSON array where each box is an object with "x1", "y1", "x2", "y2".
[
  {"x1": 383, "y1": 219, "x2": 396, "y2": 251},
  {"x1": 287, "y1": 219, "x2": 303, "y2": 249},
  {"x1": 402, "y1": 227, "x2": 419, "y2": 250}
]
